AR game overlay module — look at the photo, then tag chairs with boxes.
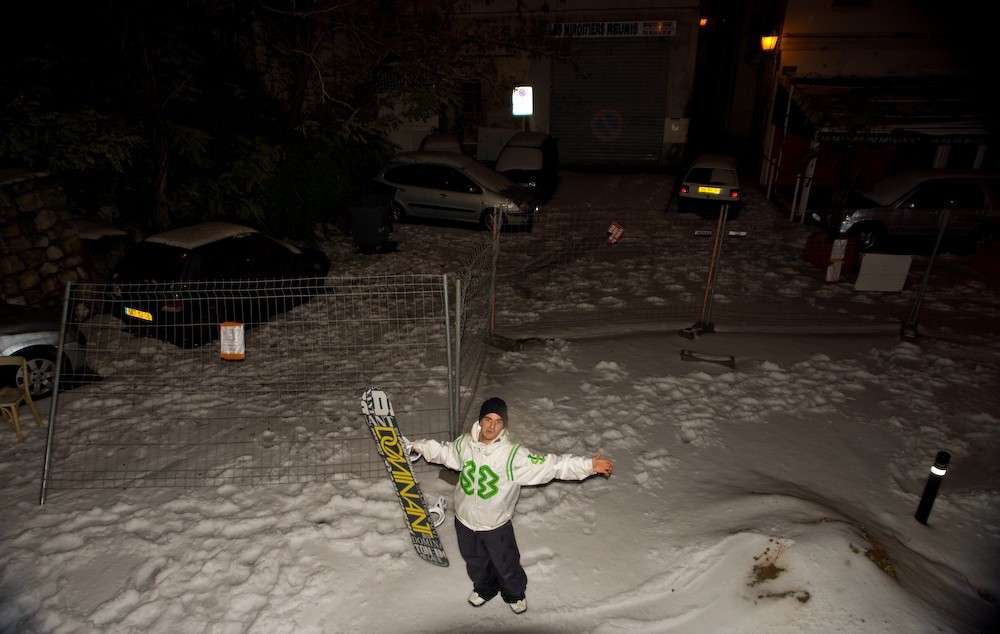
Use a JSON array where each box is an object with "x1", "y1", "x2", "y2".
[{"x1": 0, "y1": 356, "x2": 42, "y2": 443}]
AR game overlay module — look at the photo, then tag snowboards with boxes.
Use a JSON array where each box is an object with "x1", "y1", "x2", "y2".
[{"x1": 360, "y1": 386, "x2": 449, "y2": 567}]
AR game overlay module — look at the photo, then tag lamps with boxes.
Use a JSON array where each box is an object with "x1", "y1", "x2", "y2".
[
  {"x1": 930, "y1": 451, "x2": 951, "y2": 477},
  {"x1": 760, "y1": 34, "x2": 779, "y2": 54}
]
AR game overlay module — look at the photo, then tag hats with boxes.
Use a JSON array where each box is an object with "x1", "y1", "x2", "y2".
[{"x1": 479, "y1": 397, "x2": 508, "y2": 429}]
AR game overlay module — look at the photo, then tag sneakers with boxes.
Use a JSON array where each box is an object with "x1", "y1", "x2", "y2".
[
  {"x1": 510, "y1": 598, "x2": 527, "y2": 614},
  {"x1": 468, "y1": 591, "x2": 485, "y2": 607}
]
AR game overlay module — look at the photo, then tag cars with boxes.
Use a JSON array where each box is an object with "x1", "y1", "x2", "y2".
[
  {"x1": 0, "y1": 299, "x2": 88, "y2": 403},
  {"x1": 674, "y1": 153, "x2": 745, "y2": 222},
  {"x1": 810, "y1": 165, "x2": 1000, "y2": 255},
  {"x1": 375, "y1": 149, "x2": 539, "y2": 234},
  {"x1": 102, "y1": 221, "x2": 330, "y2": 351}
]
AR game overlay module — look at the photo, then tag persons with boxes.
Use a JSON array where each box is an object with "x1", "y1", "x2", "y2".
[{"x1": 399, "y1": 396, "x2": 613, "y2": 615}]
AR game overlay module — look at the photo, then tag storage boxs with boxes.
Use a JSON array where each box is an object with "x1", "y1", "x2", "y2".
[{"x1": 803, "y1": 231, "x2": 858, "y2": 274}]
